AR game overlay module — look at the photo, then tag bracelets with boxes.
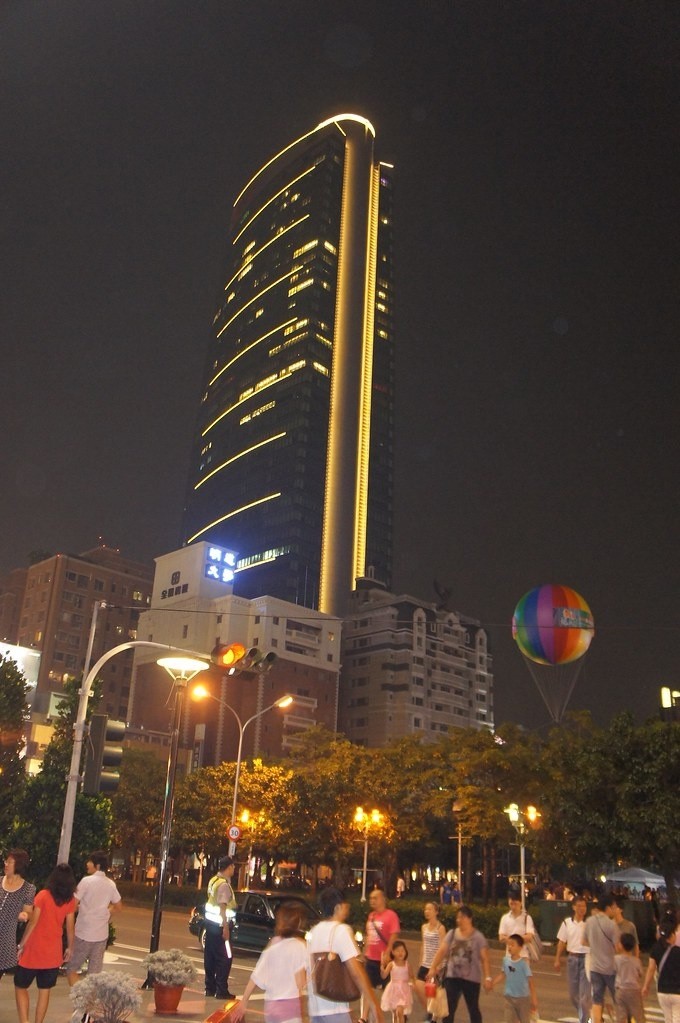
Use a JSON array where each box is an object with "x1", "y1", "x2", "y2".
[
  {"x1": 17, "y1": 945, "x2": 23, "y2": 949},
  {"x1": 485, "y1": 977, "x2": 492, "y2": 981}
]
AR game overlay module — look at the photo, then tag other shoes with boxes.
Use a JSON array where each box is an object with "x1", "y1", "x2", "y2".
[
  {"x1": 357, "y1": 1017, "x2": 368, "y2": 1023},
  {"x1": 206, "y1": 987, "x2": 217, "y2": 996},
  {"x1": 215, "y1": 990, "x2": 235, "y2": 999}
]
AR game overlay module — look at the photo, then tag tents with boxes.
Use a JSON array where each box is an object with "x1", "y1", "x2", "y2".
[{"x1": 607, "y1": 867, "x2": 667, "y2": 900}]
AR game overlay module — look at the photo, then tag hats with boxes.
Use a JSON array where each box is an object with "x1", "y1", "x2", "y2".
[{"x1": 220, "y1": 857, "x2": 237, "y2": 868}]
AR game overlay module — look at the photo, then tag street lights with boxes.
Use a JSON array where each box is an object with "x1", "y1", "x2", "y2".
[
  {"x1": 240, "y1": 809, "x2": 265, "y2": 890},
  {"x1": 504, "y1": 804, "x2": 541, "y2": 915},
  {"x1": 192, "y1": 686, "x2": 293, "y2": 856},
  {"x1": 354, "y1": 807, "x2": 384, "y2": 902},
  {"x1": 141, "y1": 656, "x2": 210, "y2": 991}
]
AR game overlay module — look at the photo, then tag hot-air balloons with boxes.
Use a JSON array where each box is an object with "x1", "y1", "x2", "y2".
[{"x1": 512, "y1": 585, "x2": 595, "y2": 726}]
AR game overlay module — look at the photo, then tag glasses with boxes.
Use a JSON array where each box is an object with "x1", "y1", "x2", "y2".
[{"x1": 4, "y1": 859, "x2": 19, "y2": 868}]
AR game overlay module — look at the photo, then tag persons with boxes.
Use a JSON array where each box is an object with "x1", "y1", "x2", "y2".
[
  {"x1": 380, "y1": 940, "x2": 417, "y2": 1023},
  {"x1": 491, "y1": 934, "x2": 538, "y2": 1023},
  {"x1": 509, "y1": 877, "x2": 667, "y2": 904},
  {"x1": 304, "y1": 887, "x2": 385, "y2": 1023},
  {"x1": 439, "y1": 879, "x2": 463, "y2": 907},
  {"x1": 0, "y1": 848, "x2": 36, "y2": 980},
  {"x1": 14, "y1": 863, "x2": 77, "y2": 1023},
  {"x1": 554, "y1": 897, "x2": 680, "y2": 1023},
  {"x1": 355, "y1": 889, "x2": 400, "y2": 1023},
  {"x1": 415, "y1": 901, "x2": 447, "y2": 1023},
  {"x1": 204, "y1": 857, "x2": 239, "y2": 999},
  {"x1": 428, "y1": 906, "x2": 493, "y2": 1023},
  {"x1": 65, "y1": 851, "x2": 123, "y2": 1023},
  {"x1": 395, "y1": 873, "x2": 406, "y2": 899},
  {"x1": 230, "y1": 901, "x2": 307, "y2": 1023},
  {"x1": 498, "y1": 893, "x2": 535, "y2": 970},
  {"x1": 145, "y1": 862, "x2": 156, "y2": 887}
]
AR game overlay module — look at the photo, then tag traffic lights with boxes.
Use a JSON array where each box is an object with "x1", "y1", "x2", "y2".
[
  {"x1": 211, "y1": 642, "x2": 279, "y2": 673},
  {"x1": 82, "y1": 713, "x2": 126, "y2": 797}
]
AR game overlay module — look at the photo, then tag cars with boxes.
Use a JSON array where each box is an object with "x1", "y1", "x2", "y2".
[
  {"x1": 279, "y1": 875, "x2": 311, "y2": 891},
  {"x1": 187, "y1": 890, "x2": 365, "y2": 953}
]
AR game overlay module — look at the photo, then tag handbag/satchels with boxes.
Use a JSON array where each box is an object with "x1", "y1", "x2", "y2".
[
  {"x1": 312, "y1": 924, "x2": 362, "y2": 1002},
  {"x1": 524, "y1": 914, "x2": 543, "y2": 962},
  {"x1": 427, "y1": 984, "x2": 450, "y2": 1017}
]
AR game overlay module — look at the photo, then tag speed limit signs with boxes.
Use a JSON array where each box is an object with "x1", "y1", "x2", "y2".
[{"x1": 227, "y1": 825, "x2": 242, "y2": 841}]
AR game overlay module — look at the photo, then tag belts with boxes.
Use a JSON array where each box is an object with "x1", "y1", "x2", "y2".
[{"x1": 569, "y1": 952, "x2": 586, "y2": 958}]
